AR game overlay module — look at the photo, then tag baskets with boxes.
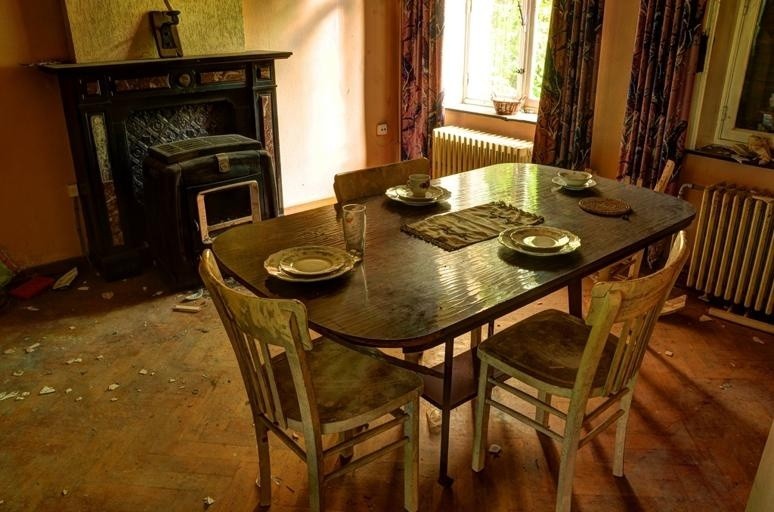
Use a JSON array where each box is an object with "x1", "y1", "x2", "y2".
[{"x1": 489, "y1": 92, "x2": 528, "y2": 115}]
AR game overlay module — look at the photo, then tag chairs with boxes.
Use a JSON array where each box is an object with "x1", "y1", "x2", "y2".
[
  {"x1": 472, "y1": 229, "x2": 691, "y2": 512},
  {"x1": 333, "y1": 157, "x2": 430, "y2": 203},
  {"x1": 585, "y1": 159, "x2": 675, "y2": 281},
  {"x1": 198, "y1": 249, "x2": 424, "y2": 512}
]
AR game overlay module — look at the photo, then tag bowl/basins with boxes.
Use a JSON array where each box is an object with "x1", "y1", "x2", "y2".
[{"x1": 557, "y1": 169, "x2": 592, "y2": 185}]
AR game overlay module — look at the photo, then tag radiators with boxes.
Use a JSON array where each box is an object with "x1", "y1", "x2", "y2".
[
  {"x1": 431, "y1": 124, "x2": 534, "y2": 180},
  {"x1": 685, "y1": 180, "x2": 774, "y2": 334}
]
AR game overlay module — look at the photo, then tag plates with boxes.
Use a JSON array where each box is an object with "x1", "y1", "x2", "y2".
[
  {"x1": 384, "y1": 185, "x2": 452, "y2": 205},
  {"x1": 262, "y1": 245, "x2": 354, "y2": 283},
  {"x1": 551, "y1": 177, "x2": 597, "y2": 190},
  {"x1": 495, "y1": 224, "x2": 582, "y2": 257}
]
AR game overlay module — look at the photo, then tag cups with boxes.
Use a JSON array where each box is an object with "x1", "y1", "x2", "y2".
[
  {"x1": 341, "y1": 203, "x2": 366, "y2": 261},
  {"x1": 406, "y1": 173, "x2": 431, "y2": 198}
]
